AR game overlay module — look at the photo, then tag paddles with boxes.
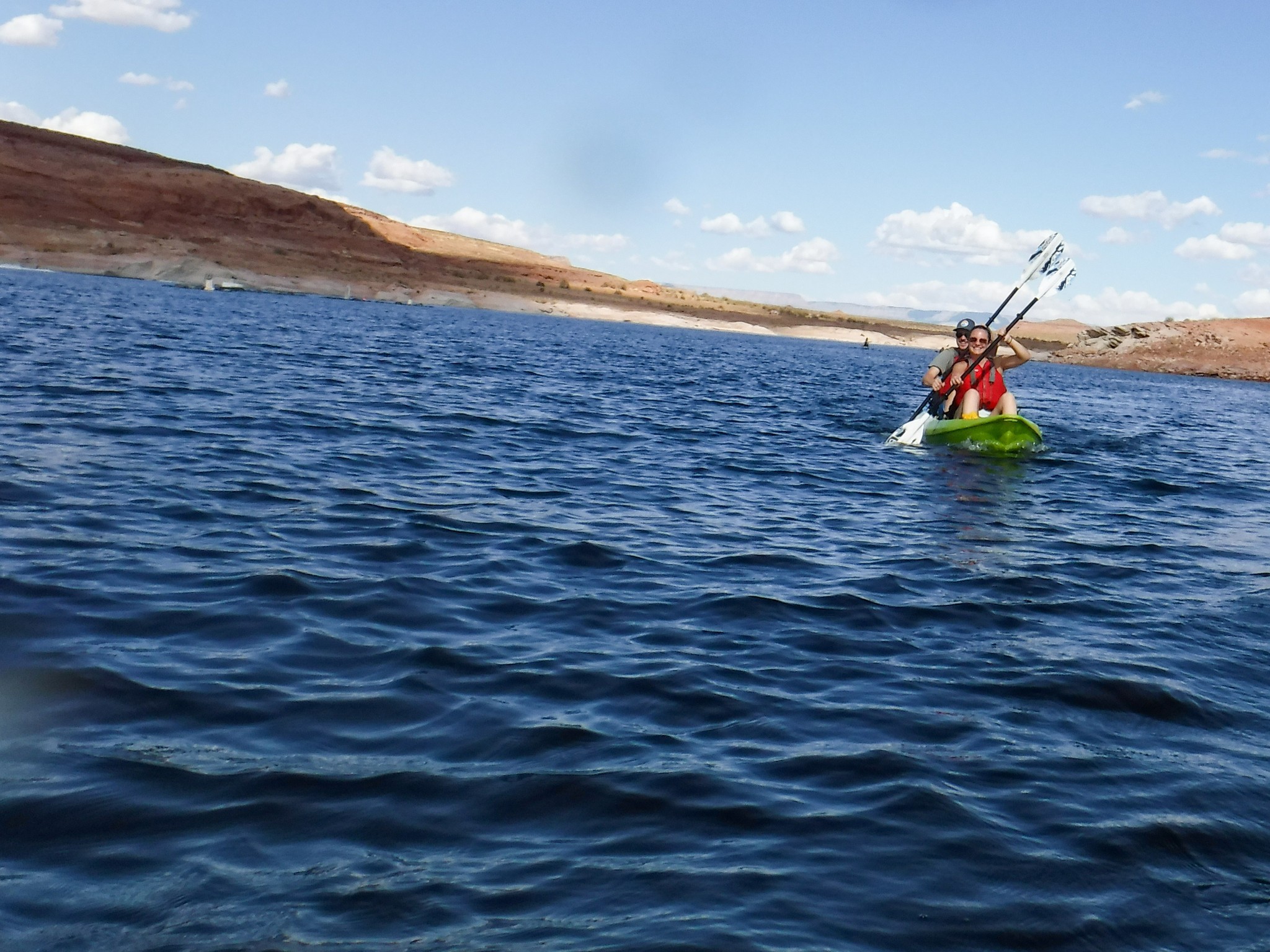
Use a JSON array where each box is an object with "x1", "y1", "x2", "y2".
[{"x1": 881, "y1": 229, "x2": 1076, "y2": 447}]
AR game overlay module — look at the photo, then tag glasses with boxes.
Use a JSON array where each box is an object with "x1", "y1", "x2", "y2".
[
  {"x1": 969, "y1": 337, "x2": 990, "y2": 343},
  {"x1": 956, "y1": 332, "x2": 970, "y2": 339}
]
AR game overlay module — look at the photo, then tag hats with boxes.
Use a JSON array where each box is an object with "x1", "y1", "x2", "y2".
[{"x1": 953, "y1": 319, "x2": 975, "y2": 332}]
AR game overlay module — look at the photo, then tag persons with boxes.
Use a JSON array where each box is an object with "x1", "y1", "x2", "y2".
[
  {"x1": 863, "y1": 337, "x2": 869, "y2": 347},
  {"x1": 921, "y1": 318, "x2": 1031, "y2": 420}
]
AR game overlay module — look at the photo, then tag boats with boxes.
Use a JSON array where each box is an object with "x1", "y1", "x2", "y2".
[
  {"x1": 863, "y1": 340, "x2": 869, "y2": 349},
  {"x1": 914, "y1": 410, "x2": 1043, "y2": 459}
]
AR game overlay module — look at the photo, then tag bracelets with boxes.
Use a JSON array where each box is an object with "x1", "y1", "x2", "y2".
[{"x1": 1006, "y1": 338, "x2": 1012, "y2": 344}]
